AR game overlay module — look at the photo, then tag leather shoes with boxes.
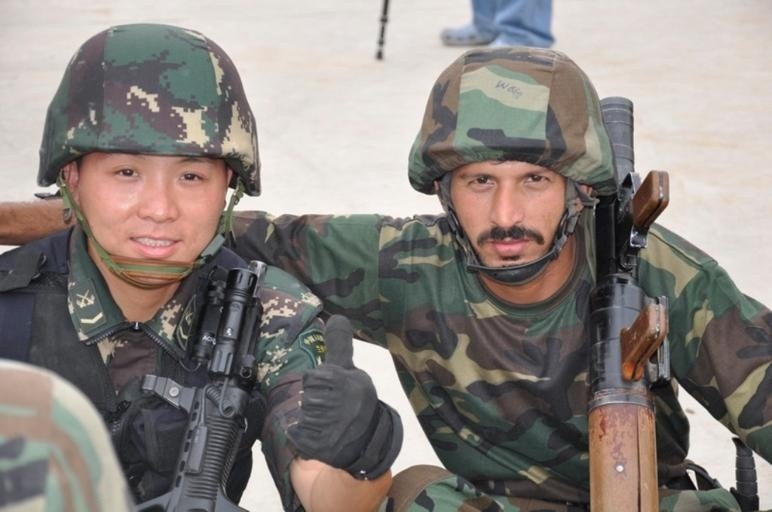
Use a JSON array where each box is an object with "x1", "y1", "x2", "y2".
[{"x1": 442, "y1": 22, "x2": 487, "y2": 45}]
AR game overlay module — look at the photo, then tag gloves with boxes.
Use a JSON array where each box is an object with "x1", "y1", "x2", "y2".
[{"x1": 283, "y1": 312, "x2": 403, "y2": 480}]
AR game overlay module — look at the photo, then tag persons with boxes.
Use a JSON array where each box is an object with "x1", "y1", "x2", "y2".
[
  {"x1": 0, "y1": 47, "x2": 772, "y2": 512},
  {"x1": 0, "y1": 24, "x2": 402, "y2": 512},
  {"x1": 439, "y1": 0, "x2": 555, "y2": 51}
]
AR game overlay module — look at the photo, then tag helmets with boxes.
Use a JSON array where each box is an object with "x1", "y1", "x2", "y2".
[
  {"x1": 38, "y1": 24, "x2": 260, "y2": 197},
  {"x1": 407, "y1": 45, "x2": 618, "y2": 196},
  {"x1": 0, "y1": 360, "x2": 132, "y2": 512}
]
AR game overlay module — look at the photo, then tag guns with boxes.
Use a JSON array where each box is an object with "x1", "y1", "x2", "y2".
[
  {"x1": 588, "y1": 96, "x2": 672, "y2": 511},
  {"x1": 133, "y1": 261, "x2": 267, "y2": 512}
]
